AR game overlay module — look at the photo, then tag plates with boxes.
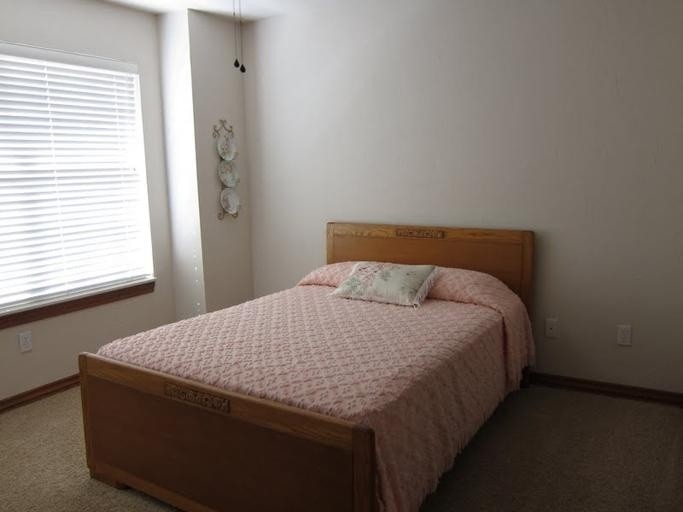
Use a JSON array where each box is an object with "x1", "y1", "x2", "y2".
[
  {"x1": 217, "y1": 132, "x2": 237, "y2": 161},
  {"x1": 220, "y1": 188, "x2": 242, "y2": 215},
  {"x1": 218, "y1": 160, "x2": 240, "y2": 188}
]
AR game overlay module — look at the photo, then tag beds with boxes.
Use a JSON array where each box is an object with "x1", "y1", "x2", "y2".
[{"x1": 78, "y1": 222, "x2": 535, "y2": 512}]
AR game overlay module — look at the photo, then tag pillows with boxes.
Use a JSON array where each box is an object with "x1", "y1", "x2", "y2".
[{"x1": 329, "y1": 262, "x2": 439, "y2": 306}]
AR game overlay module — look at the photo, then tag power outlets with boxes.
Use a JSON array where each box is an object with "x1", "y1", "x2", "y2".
[{"x1": 19, "y1": 331, "x2": 32, "y2": 351}]
